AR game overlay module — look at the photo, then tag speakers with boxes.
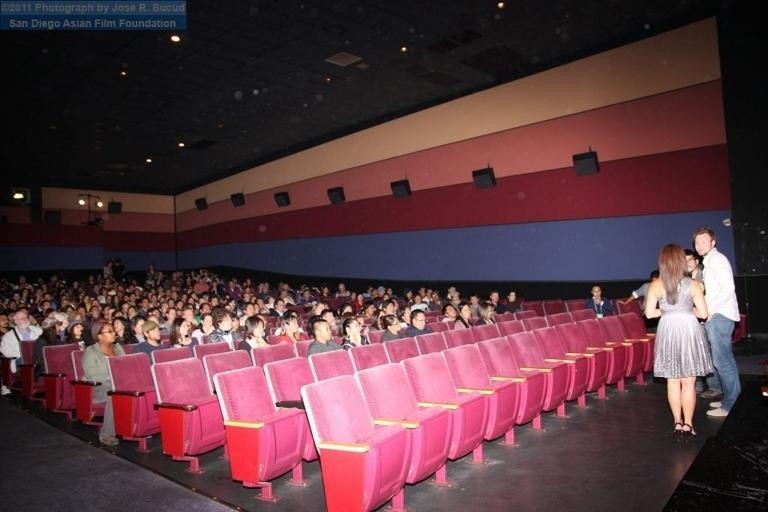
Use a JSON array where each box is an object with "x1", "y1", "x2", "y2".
[
  {"x1": 45, "y1": 211, "x2": 61, "y2": 224},
  {"x1": 230, "y1": 192, "x2": 245, "y2": 206},
  {"x1": 273, "y1": 190, "x2": 291, "y2": 208},
  {"x1": 473, "y1": 166, "x2": 496, "y2": 189},
  {"x1": 573, "y1": 150, "x2": 600, "y2": 176},
  {"x1": 194, "y1": 197, "x2": 208, "y2": 209},
  {"x1": 326, "y1": 186, "x2": 344, "y2": 204},
  {"x1": 390, "y1": 178, "x2": 411, "y2": 198},
  {"x1": 108, "y1": 202, "x2": 122, "y2": 214}
]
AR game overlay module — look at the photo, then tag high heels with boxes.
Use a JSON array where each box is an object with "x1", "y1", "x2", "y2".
[
  {"x1": 672, "y1": 423, "x2": 682, "y2": 444},
  {"x1": 682, "y1": 424, "x2": 697, "y2": 449}
]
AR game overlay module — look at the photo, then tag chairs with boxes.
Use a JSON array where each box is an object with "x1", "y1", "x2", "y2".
[
  {"x1": 300, "y1": 374, "x2": 418, "y2": 512},
  {"x1": 213, "y1": 366, "x2": 305, "y2": 501}
]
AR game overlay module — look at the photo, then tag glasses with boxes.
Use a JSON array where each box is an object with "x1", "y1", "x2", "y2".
[{"x1": 102, "y1": 329, "x2": 115, "y2": 333}]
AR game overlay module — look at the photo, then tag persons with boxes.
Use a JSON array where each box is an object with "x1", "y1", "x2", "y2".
[
  {"x1": 585, "y1": 286, "x2": 615, "y2": 318},
  {"x1": 81, "y1": 320, "x2": 126, "y2": 446},
  {"x1": 1, "y1": 259, "x2": 524, "y2": 395},
  {"x1": 618, "y1": 226, "x2": 741, "y2": 445}
]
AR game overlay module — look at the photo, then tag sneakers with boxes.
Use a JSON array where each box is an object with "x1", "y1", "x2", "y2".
[
  {"x1": 706, "y1": 408, "x2": 729, "y2": 417},
  {"x1": 709, "y1": 401, "x2": 721, "y2": 408},
  {"x1": 699, "y1": 388, "x2": 723, "y2": 399},
  {"x1": 1, "y1": 386, "x2": 11, "y2": 396},
  {"x1": 100, "y1": 436, "x2": 119, "y2": 446}
]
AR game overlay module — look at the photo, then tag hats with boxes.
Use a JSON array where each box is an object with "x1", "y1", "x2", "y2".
[
  {"x1": 141, "y1": 321, "x2": 159, "y2": 331},
  {"x1": 42, "y1": 317, "x2": 62, "y2": 329}
]
